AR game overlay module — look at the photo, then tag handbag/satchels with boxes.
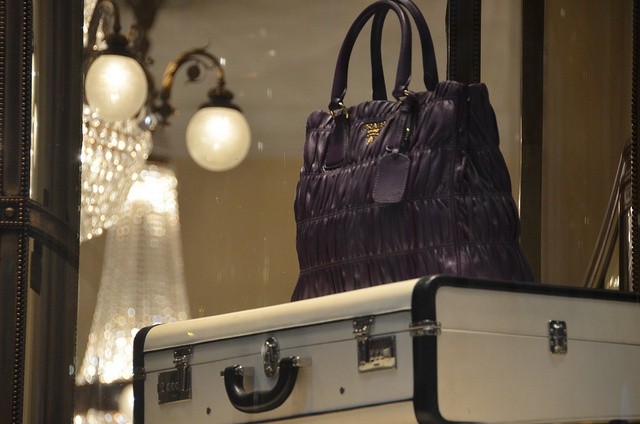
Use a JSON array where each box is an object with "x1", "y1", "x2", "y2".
[{"x1": 288, "y1": 1, "x2": 536, "y2": 300}]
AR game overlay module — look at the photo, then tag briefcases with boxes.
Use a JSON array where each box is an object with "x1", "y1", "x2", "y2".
[{"x1": 133, "y1": 276, "x2": 639, "y2": 423}]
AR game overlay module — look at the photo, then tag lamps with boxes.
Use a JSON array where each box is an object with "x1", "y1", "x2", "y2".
[{"x1": 79, "y1": 0, "x2": 253, "y2": 246}]
